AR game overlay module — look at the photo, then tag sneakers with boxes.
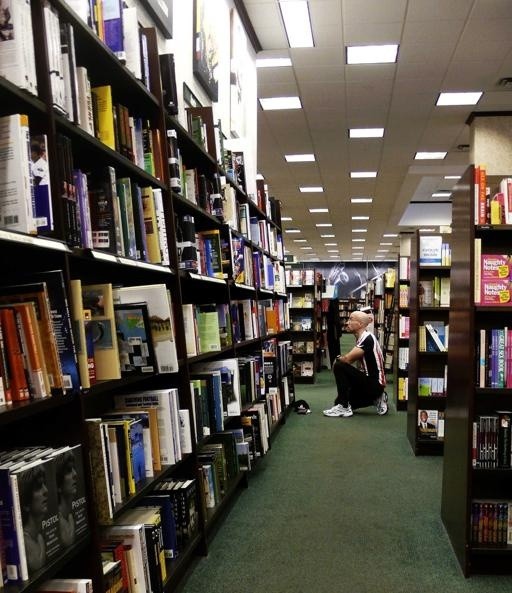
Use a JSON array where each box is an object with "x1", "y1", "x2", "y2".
[
  {"x1": 323, "y1": 401, "x2": 353, "y2": 417},
  {"x1": 374, "y1": 392, "x2": 388, "y2": 415}
]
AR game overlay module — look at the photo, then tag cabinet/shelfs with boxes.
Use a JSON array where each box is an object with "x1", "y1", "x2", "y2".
[
  {"x1": 374, "y1": 110, "x2": 512, "y2": 581},
  {"x1": 3, "y1": 0, "x2": 333, "y2": 593}
]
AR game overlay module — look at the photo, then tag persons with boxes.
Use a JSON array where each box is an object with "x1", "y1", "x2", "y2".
[
  {"x1": 333, "y1": 272, "x2": 362, "y2": 299},
  {"x1": 323, "y1": 311, "x2": 388, "y2": 417}
]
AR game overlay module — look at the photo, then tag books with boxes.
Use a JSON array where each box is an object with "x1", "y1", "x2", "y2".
[
  {"x1": 0, "y1": 2, "x2": 296, "y2": 593},
  {"x1": 339, "y1": 302, "x2": 357, "y2": 333},
  {"x1": 284, "y1": 270, "x2": 333, "y2": 384},
  {"x1": 365, "y1": 257, "x2": 413, "y2": 400},
  {"x1": 474, "y1": 166, "x2": 512, "y2": 545},
  {"x1": 419, "y1": 236, "x2": 452, "y2": 439}
]
